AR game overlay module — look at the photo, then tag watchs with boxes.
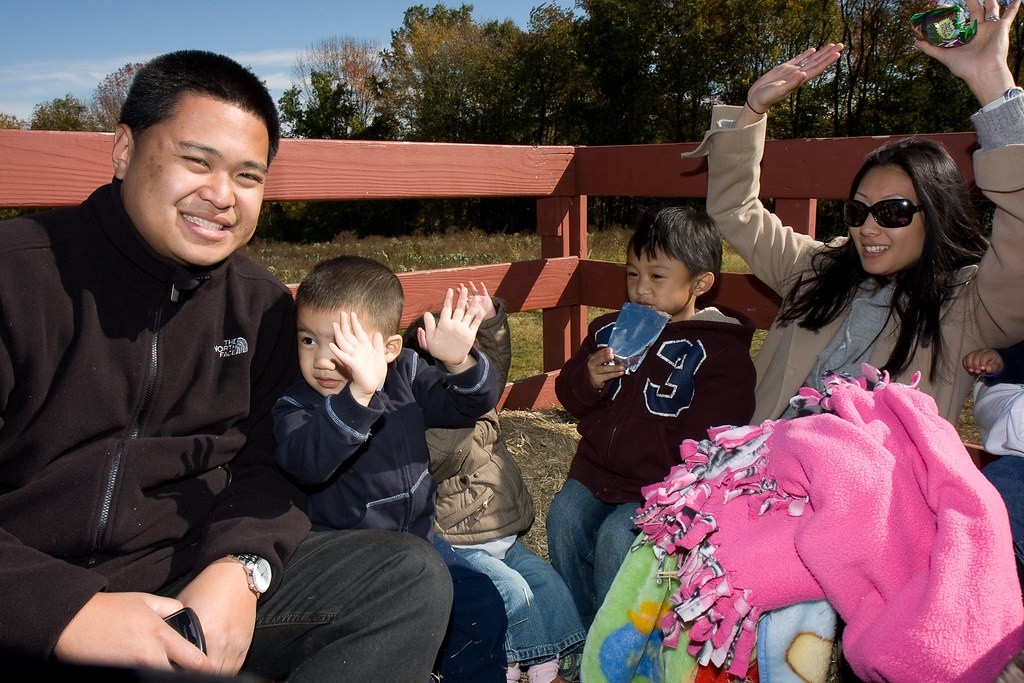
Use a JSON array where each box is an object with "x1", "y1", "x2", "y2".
[
  {"x1": 980, "y1": 86, "x2": 1023, "y2": 114},
  {"x1": 228, "y1": 550, "x2": 272, "y2": 596}
]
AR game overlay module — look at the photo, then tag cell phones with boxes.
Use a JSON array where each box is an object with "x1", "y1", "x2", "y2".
[{"x1": 161, "y1": 606, "x2": 207, "y2": 672}]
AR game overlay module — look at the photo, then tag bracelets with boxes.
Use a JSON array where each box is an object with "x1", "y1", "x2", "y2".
[{"x1": 747, "y1": 100, "x2": 766, "y2": 114}]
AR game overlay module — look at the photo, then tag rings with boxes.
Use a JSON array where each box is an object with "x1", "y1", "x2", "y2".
[
  {"x1": 987, "y1": 15, "x2": 1000, "y2": 21},
  {"x1": 799, "y1": 61, "x2": 804, "y2": 67}
]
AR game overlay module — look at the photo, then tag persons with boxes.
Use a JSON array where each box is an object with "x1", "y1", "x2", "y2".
[
  {"x1": 681, "y1": 1, "x2": 1023, "y2": 682},
  {"x1": 270, "y1": 255, "x2": 507, "y2": 683},
  {"x1": 0, "y1": 49, "x2": 454, "y2": 682},
  {"x1": 405, "y1": 283, "x2": 587, "y2": 683},
  {"x1": 548, "y1": 206, "x2": 756, "y2": 683}
]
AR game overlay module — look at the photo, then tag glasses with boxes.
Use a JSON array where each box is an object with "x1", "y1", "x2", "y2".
[{"x1": 843, "y1": 198, "x2": 923, "y2": 229}]
